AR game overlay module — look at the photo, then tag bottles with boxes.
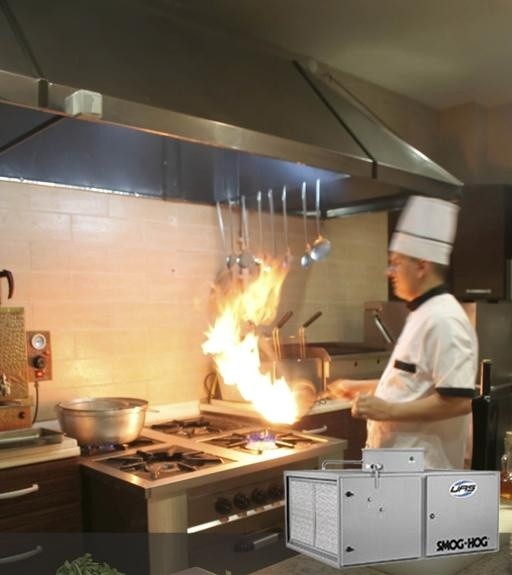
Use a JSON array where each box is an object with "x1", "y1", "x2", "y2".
[
  {"x1": 499, "y1": 428, "x2": 512, "y2": 509},
  {"x1": 473, "y1": 358, "x2": 501, "y2": 473}
]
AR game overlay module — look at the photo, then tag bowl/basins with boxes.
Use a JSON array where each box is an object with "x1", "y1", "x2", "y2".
[{"x1": 54, "y1": 394, "x2": 147, "y2": 446}]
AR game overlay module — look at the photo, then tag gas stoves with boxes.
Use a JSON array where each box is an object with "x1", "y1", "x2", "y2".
[{"x1": 51, "y1": 413, "x2": 347, "y2": 533}]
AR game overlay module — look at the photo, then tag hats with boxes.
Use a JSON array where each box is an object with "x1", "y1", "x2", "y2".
[{"x1": 389, "y1": 195, "x2": 462, "y2": 265}]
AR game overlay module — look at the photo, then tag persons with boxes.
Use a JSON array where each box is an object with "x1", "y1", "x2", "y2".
[{"x1": 327, "y1": 251, "x2": 478, "y2": 470}]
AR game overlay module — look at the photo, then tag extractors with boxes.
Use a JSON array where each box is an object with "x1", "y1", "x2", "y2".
[{"x1": 0, "y1": 1, "x2": 462, "y2": 217}]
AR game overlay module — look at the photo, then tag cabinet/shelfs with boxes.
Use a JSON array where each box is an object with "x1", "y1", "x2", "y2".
[
  {"x1": 0, "y1": 457, "x2": 83, "y2": 574},
  {"x1": 289, "y1": 407, "x2": 367, "y2": 471}
]
